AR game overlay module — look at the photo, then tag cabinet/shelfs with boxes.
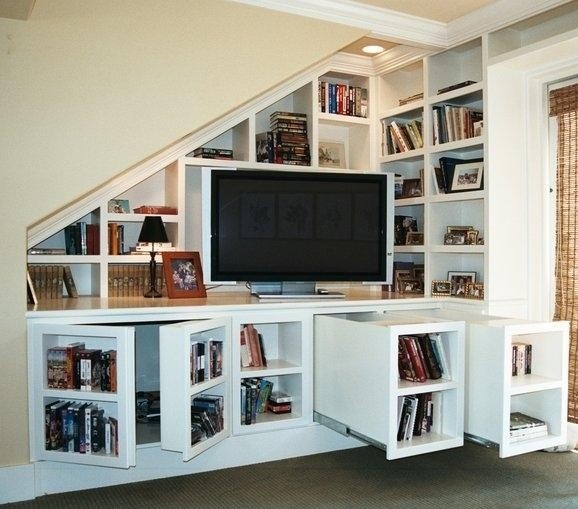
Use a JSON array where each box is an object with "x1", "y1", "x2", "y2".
[
  {"x1": 313, "y1": 306, "x2": 466, "y2": 463},
  {"x1": 21, "y1": 304, "x2": 232, "y2": 498},
  {"x1": 397, "y1": 305, "x2": 570, "y2": 461},
  {"x1": 26, "y1": 31, "x2": 518, "y2": 300},
  {"x1": 232, "y1": 304, "x2": 312, "y2": 468}
]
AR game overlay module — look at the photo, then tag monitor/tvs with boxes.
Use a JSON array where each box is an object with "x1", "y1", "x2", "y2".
[{"x1": 202, "y1": 165, "x2": 395, "y2": 300}]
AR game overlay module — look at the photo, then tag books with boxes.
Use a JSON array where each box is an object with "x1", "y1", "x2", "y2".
[
  {"x1": 190, "y1": 324, "x2": 293, "y2": 446},
  {"x1": 26, "y1": 205, "x2": 178, "y2": 301},
  {"x1": 45, "y1": 342, "x2": 119, "y2": 458},
  {"x1": 510, "y1": 412, "x2": 548, "y2": 444},
  {"x1": 512, "y1": 343, "x2": 532, "y2": 377},
  {"x1": 396, "y1": 333, "x2": 451, "y2": 444},
  {"x1": 186, "y1": 80, "x2": 484, "y2": 201}
]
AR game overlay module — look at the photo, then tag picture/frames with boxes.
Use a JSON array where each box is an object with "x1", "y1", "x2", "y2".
[
  {"x1": 161, "y1": 251, "x2": 207, "y2": 299},
  {"x1": 448, "y1": 160, "x2": 483, "y2": 191},
  {"x1": 446, "y1": 270, "x2": 475, "y2": 297}
]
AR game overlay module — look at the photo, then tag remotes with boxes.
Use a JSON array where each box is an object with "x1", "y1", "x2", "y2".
[{"x1": 318, "y1": 288, "x2": 330, "y2": 295}]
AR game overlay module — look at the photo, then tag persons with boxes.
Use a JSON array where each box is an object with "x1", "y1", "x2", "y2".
[{"x1": 172, "y1": 260, "x2": 197, "y2": 291}]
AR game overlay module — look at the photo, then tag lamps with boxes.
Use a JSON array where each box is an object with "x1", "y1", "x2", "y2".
[{"x1": 139, "y1": 216, "x2": 169, "y2": 299}]
